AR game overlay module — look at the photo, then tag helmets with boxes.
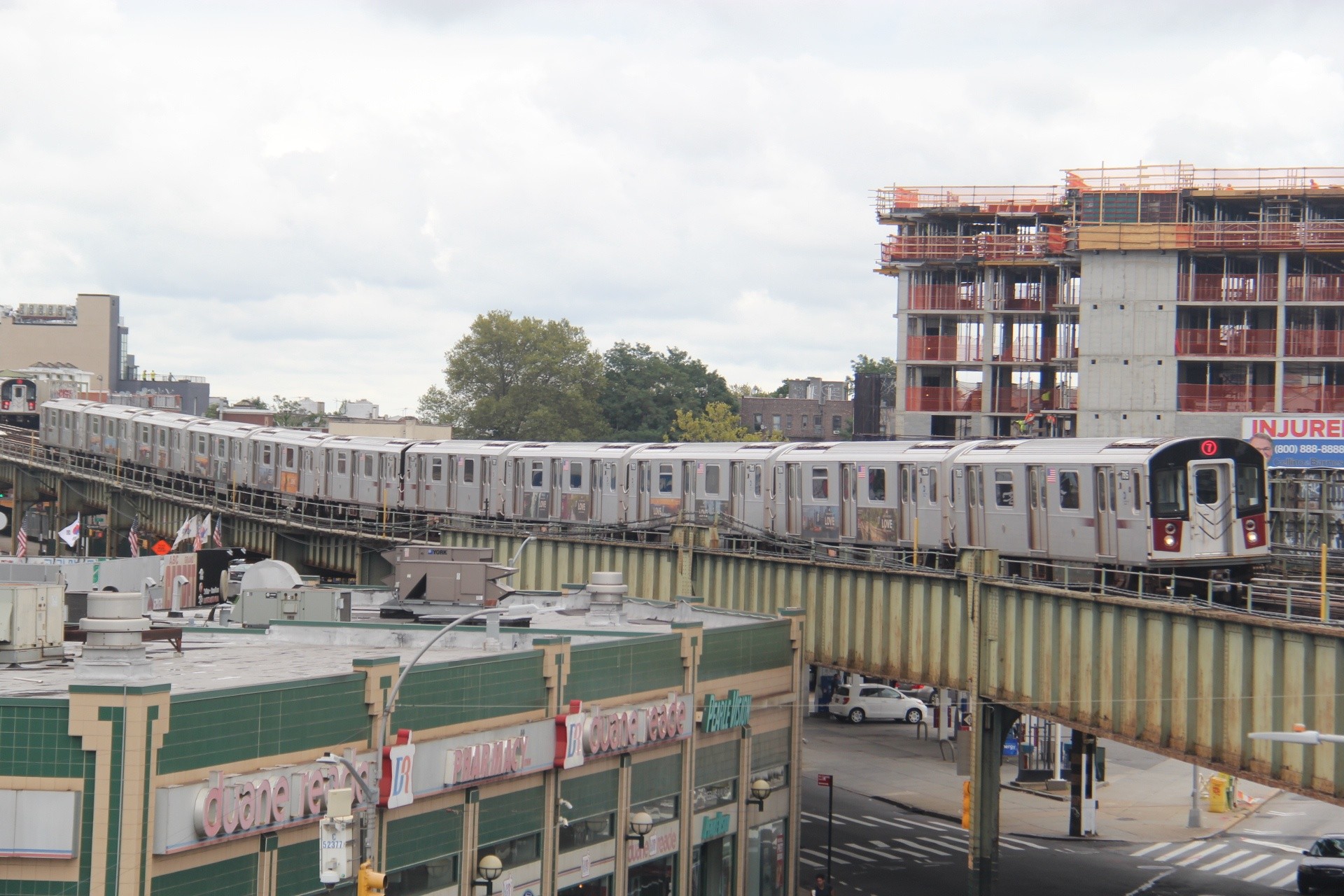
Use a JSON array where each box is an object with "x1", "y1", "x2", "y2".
[{"x1": 1030, "y1": 409, "x2": 1033, "y2": 412}]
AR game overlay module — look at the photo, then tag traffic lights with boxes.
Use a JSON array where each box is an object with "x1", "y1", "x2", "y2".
[
  {"x1": 356, "y1": 859, "x2": 389, "y2": 896},
  {"x1": 962, "y1": 782, "x2": 971, "y2": 830}
]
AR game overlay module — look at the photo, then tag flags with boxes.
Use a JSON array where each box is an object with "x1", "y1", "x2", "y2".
[
  {"x1": 57, "y1": 511, "x2": 79, "y2": 547},
  {"x1": 171, "y1": 512, "x2": 221, "y2": 551},
  {"x1": 128, "y1": 513, "x2": 139, "y2": 557},
  {"x1": 15, "y1": 514, "x2": 26, "y2": 557}
]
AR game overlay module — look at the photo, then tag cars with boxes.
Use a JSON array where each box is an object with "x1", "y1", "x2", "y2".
[
  {"x1": 1297, "y1": 833, "x2": 1344, "y2": 894},
  {"x1": 894, "y1": 681, "x2": 940, "y2": 708}
]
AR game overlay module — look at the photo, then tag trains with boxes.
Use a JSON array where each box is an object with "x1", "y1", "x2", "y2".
[
  {"x1": 1, "y1": 379, "x2": 43, "y2": 428},
  {"x1": 33, "y1": 395, "x2": 1280, "y2": 582}
]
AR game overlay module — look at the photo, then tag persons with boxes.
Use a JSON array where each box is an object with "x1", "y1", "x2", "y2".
[
  {"x1": 829, "y1": 673, "x2": 841, "y2": 720},
  {"x1": 810, "y1": 872, "x2": 835, "y2": 896},
  {"x1": 1249, "y1": 432, "x2": 1273, "y2": 463}
]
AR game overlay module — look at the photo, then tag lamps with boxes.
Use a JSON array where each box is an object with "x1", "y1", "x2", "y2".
[
  {"x1": 746, "y1": 779, "x2": 771, "y2": 812},
  {"x1": 472, "y1": 854, "x2": 503, "y2": 896},
  {"x1": 625, "y1": 812, "x2": 653, "y2": 849}
]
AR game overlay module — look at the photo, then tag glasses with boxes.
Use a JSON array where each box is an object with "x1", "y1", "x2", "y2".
[{"x1": 1256, "y1": 447, "x2": 1272, "y2": 453}]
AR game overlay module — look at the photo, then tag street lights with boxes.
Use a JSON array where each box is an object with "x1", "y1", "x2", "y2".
[{"x1": 317, "y1": 605, "x2": 541, "y2": 869}]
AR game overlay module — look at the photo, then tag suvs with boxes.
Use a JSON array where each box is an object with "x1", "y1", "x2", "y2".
[{"x1": 828, "y1": 683, "x2": 929, "y2": 726}]
